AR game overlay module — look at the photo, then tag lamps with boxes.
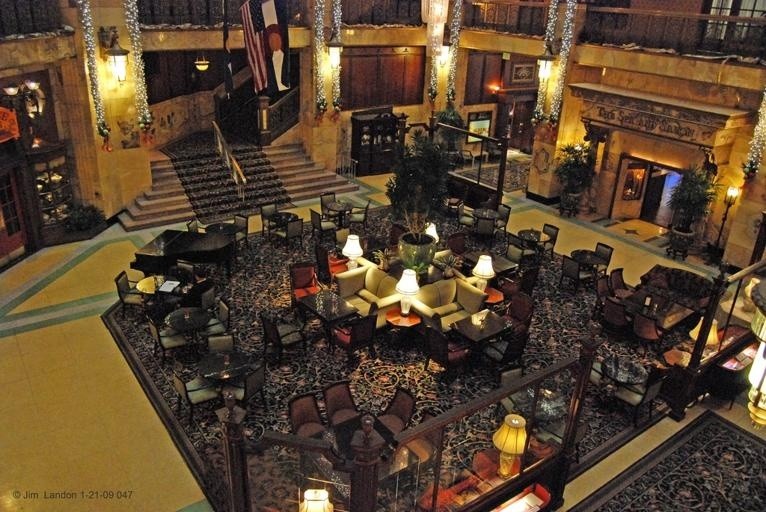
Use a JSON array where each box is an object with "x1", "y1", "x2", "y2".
[
  {"x1": 747, "y1": 277, "x2": 766, "y2": 433},
  {"x1": 194, "y1": 48, "x2": 210, "y2": 72},
  {"x1": 2, "y1": 75, "x2": 42, "y2": 97},
  {"x1": 97, "y1": 21, "x2": 132, "y2": 87},
  {"x1": 324, "y1": 22, "x2": 345, "y2": 69},
  {"x1": 536, "y1": 38, "x2": 561, "y2": 83},
  {"x1": 742, "y1": 278, "x2": 760, "y2": 312},
  {"x1": 439, "y1": 22, "x2": 452, "y2": 66},
  {"x1": 711, "y1": 181, "x2": 742, "y2": 254}
]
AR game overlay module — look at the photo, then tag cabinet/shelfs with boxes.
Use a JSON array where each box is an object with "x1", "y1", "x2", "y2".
[
  {"x1": 699, "y1": 340, "x2": 758, "y2": 411},
  {"x1": 350, "y1": 105, "x2": 409, "y2": 175},
  {"x1": 27, "y1": 145, "x2": 75, "y2": 246}
]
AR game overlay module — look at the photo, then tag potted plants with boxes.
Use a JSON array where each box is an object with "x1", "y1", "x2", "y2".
[
  {"x1": 665, "y1": 161, "x2": 718, "y2": 251},
  {"x1": 435, "y1": 108, "x2": 465, "y2": 165},
  {"x1": 554, "y1": 141, "x2": 599, "y2": 217},
  {"x1": 387, "y1": 128, "x2": 452, "y2": 269}
]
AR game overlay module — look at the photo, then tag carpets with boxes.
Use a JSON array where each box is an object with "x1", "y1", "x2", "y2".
[{"x1": 565, "y1": 410, "x2": 766, "y2": 510}]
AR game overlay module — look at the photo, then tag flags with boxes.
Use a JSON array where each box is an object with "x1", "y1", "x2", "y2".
[
  {"x1": 221, "y1": 0, "x2": 234, "y2": 95},
  {"x1": 237, "y1": 0, "x2": 293, "y2": 94}
]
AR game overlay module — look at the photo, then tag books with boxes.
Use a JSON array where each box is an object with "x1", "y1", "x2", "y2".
[{"x1": 158, "y1": 280, "x2": 180, "y2": 293}]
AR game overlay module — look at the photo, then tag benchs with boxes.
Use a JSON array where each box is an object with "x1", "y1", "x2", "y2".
[{"x1": 462, "y1": 150, "x2": 489, "y2": 168}]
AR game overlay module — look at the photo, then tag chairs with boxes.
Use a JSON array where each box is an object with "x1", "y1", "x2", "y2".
[{"x1": 100, "y1": 192, "x2": 729, "y2": 512}]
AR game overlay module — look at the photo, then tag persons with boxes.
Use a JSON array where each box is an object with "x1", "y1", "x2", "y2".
[{"x1": 183, "y1": 270, "x2": 216, "y2": 307}]
